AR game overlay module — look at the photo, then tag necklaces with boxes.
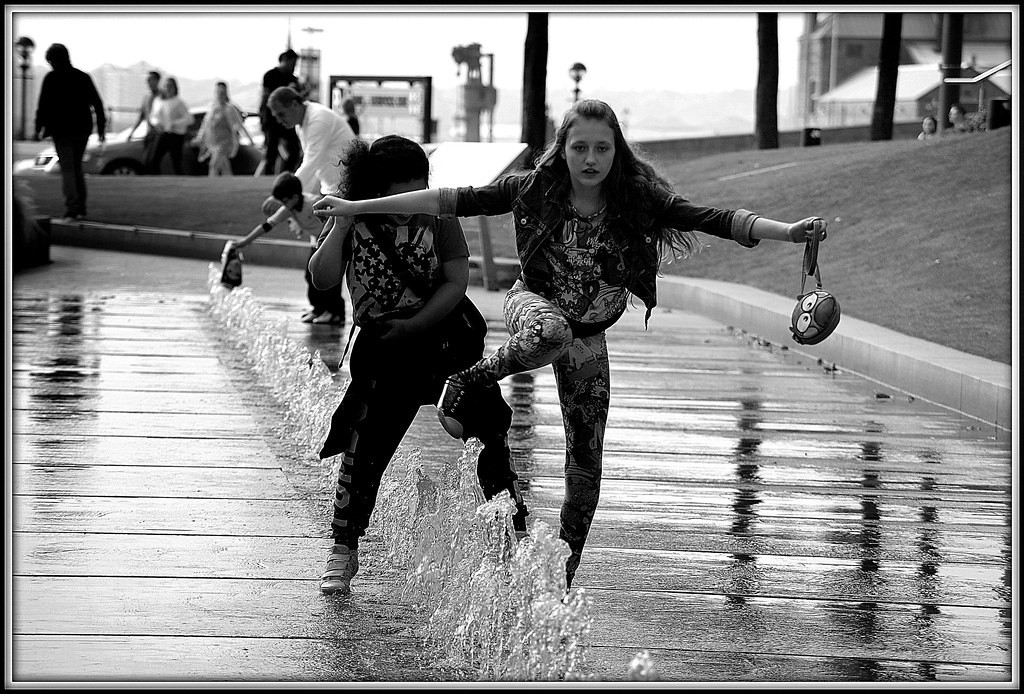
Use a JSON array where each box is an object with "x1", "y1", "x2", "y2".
[{"x1": 567, "y1": 198, "x2": 607, "y2": 220}]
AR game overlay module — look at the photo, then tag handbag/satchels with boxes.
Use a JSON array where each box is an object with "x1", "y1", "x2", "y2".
[
  {"x1": 430, "y1": 294, "x2": 487, "y2": 362},
  {"x1": 790, "y1": 218, "x2": 842, "y2": 348}
]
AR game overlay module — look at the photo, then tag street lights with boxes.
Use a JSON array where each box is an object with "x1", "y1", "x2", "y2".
[
  {"x1": 14, "y1": 37, "x2": 35, "y2": 142},
  {"x1": 569, "y1": 63, "x2": 586, "y2": 102}
]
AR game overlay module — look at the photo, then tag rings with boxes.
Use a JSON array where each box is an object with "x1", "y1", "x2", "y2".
[{"x1": 821, "y1": 232, "x2": 825, "y2": 239}]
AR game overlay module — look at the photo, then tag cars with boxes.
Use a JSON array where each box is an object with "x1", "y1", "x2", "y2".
[
  {"x1": 81, "y1": 108, "x2": 289, "y2": 177},
  {"x1": 34, "y1": 134, "x2": 100, "y2": 173}
]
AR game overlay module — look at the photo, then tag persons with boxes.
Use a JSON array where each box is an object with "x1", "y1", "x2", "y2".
[
  {"x1": 313, "y1": 100, "x2": 827, "y2": 593},
  {"x1": 308, "y1": 134, "x2": 532, "y2": 594},
  {"x1": 254, "y1": 49, "x2": 309, "y2": 176},
  {"x1": 31, "y1": 43, "x2": 106, "y2": 221},
  {"x1": 917, "y1": 104, "x2": 975, "y2": 140},
  {"x1": 128, "y1": 71, "x2": 254, "y2": 176},
  {"x1": 232, "y1": 86, "x2": 360, "y2": 326}
]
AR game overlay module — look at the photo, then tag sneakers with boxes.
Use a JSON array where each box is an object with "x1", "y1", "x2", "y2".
[
  {"x1": 319, "y1": 543, "x2": 360, "y2": 594},
  {"x1": 437, "y1": 361, "x2": 486, "y2": 441}
]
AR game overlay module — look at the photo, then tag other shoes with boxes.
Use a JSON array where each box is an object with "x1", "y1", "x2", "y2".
[{"x1": 301, "y1": 312, "x2": 346, "y2": 324}]
